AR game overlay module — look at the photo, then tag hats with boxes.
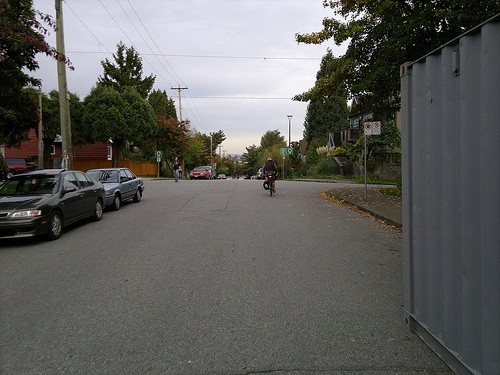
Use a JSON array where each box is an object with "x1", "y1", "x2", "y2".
[{"x1": 267, "y1": 157, "x2": 272, "y2": 161}]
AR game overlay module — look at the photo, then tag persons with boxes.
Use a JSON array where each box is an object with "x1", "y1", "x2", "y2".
[
  {"x1": 172, "y1": 156, "x2": 180, "y2": 182},
  {"x1": 263, "y1": 157, "x2": 279, "y2": 189}
]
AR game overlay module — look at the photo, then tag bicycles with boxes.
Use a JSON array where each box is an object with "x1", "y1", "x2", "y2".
[{"x1": 267, "y1": 174, "x2": 273, "y2": 197}]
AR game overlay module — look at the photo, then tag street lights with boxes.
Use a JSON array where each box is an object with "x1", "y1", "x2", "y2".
[{"x1": 287, "y1": 115, "x2": 293, "y2": 148}]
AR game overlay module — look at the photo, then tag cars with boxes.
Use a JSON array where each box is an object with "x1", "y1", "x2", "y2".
[
  {"x1": 256, "y1": 168, "x2": 262, "y2": 178},
  {"x1": 0, "y1": 168, "x2": 106, "y2": 240},
  {"x1": 218, "y1": 174, "x2": 227, "y2": 179},
  {"x1": 86, "y1": 167, "x2": 145, "y2": 212},
  {"x1": 189, "y1": 166, "x2": 218, "y2": 180}
]
